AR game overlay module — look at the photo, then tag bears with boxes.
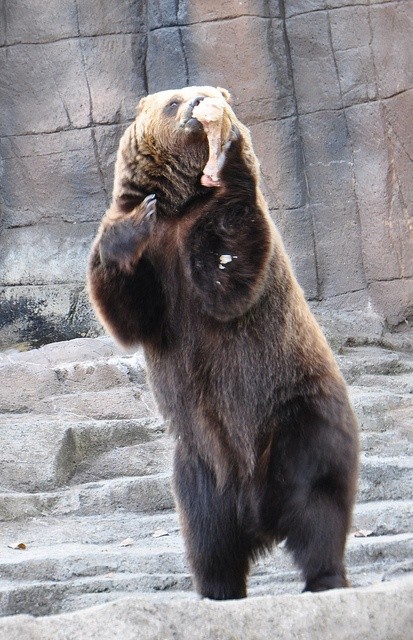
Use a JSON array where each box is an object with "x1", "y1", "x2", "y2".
[{"x1": 85, "y1": 83, "x2": 360, "y2": 601}]
